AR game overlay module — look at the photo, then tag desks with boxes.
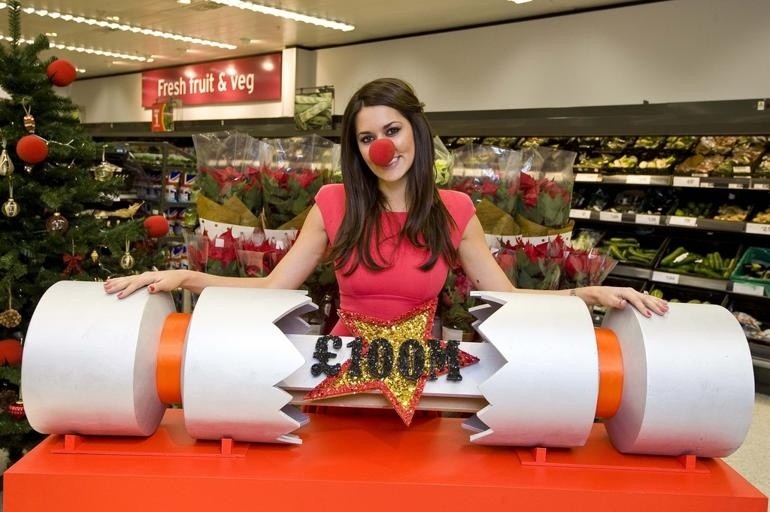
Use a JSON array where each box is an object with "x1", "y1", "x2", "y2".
[{"x1": 2, "y1": 407, "x2": 768, "y2": 512}]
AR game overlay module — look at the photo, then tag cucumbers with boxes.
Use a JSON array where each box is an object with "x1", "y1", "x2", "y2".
[{"x1": 594, "y1": 237, "x2": 735, "y2": 280}]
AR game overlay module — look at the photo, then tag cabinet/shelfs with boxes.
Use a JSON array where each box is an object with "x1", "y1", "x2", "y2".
[
  {"x1": 95, "y1": 136, "x2": 196, "y2": 270},
  {"x1": 443, "y1": 136, "x2": 768, "y2": 394}
]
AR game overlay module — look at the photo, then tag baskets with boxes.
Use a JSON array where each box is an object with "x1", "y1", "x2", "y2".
[{"x1": 731, "y1": 246, "x2": 770, "y2": 299}]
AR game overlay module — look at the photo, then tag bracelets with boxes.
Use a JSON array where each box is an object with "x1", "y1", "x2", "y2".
[{"x1": 570, "y1": 288, "x2": 576, "y2": 297}]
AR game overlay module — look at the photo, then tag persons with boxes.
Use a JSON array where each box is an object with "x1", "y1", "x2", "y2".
[{"x1": 105, "y1": 78, "x2": 669, "y2": 420}]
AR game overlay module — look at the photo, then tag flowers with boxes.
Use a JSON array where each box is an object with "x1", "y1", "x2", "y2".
[
  {"x1": 185, "y1": 164, "x2": 342, "y2": 329},
  {"x1": 433, "y1": 165, "x2": 615, "y2": 329}
]
